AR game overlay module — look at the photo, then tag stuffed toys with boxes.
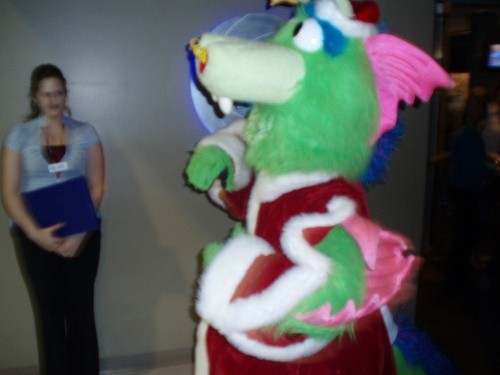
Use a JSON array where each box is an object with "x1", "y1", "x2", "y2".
[{"x1": 185, "y1": 0, "x2": 458, "y2": 375}]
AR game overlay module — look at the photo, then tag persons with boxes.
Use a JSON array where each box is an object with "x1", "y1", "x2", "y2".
[
  {"x1": 419, "y1": 74, "x2": 500, "y2": 283},
  {"x1": 0, "y1": 63, "x2": 105, "y2": 375}
]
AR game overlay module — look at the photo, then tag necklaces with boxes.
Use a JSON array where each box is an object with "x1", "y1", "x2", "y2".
[{"x1": 43, "y1": 123, "x2": 65, "y2": 181}]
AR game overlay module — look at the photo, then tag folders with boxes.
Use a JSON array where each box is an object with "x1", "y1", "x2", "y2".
[{"x1": 22, "y1": 176, "x2": 98, "y2": 239}]
